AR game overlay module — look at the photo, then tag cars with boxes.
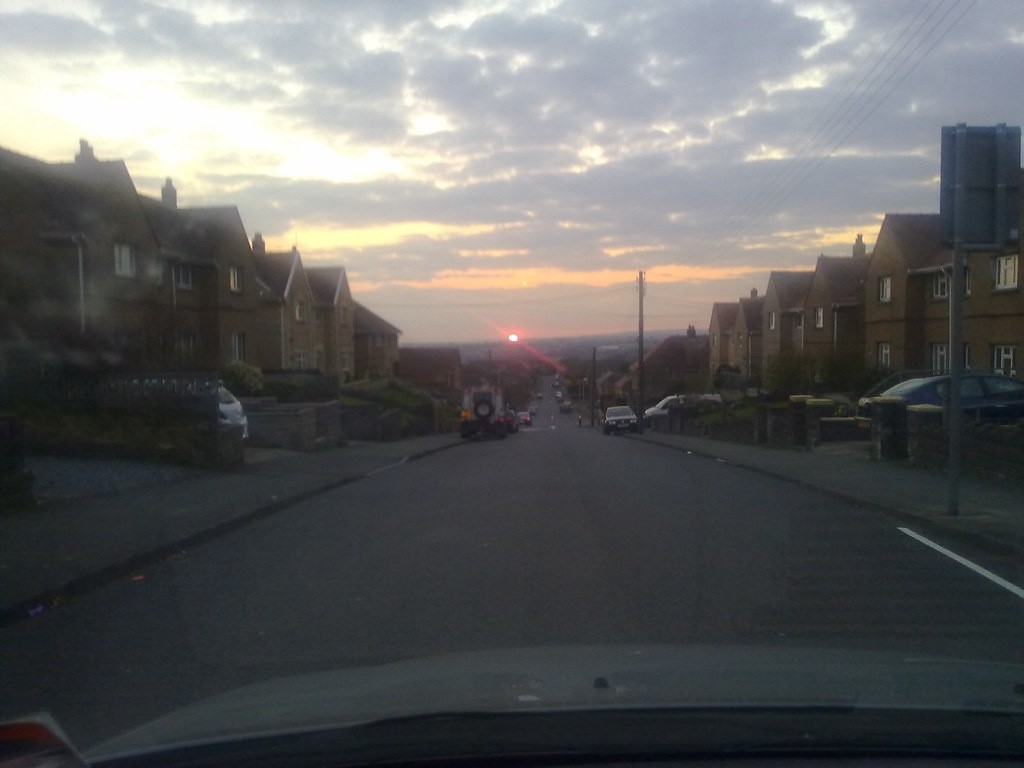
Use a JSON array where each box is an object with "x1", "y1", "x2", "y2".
[
  {"x1": 505, "y1": 409, "x2": 519, "y2": 434},
  {"x1": 853, "y1": 372, "x2": 1023, "y2": 450},
  {"x1": 602, "y1": 405, "x2": 639, "y2": 435},
  {"x1": 515, "y1": 412, "x2": 531, "y2": 426}
]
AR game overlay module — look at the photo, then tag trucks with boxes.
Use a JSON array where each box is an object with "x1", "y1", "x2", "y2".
[{"x1": 461, "y1": 386, "x2": 505, "y2": 440}]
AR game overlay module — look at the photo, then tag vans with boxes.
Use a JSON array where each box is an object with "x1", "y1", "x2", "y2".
[{"x1": 642, "y1": 392, "x2": 721, "y2": 428}]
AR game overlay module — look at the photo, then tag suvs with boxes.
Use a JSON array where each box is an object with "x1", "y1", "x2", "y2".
[{"x1": 123, "y1": 379, "x2": 248, "y2": 441}]
